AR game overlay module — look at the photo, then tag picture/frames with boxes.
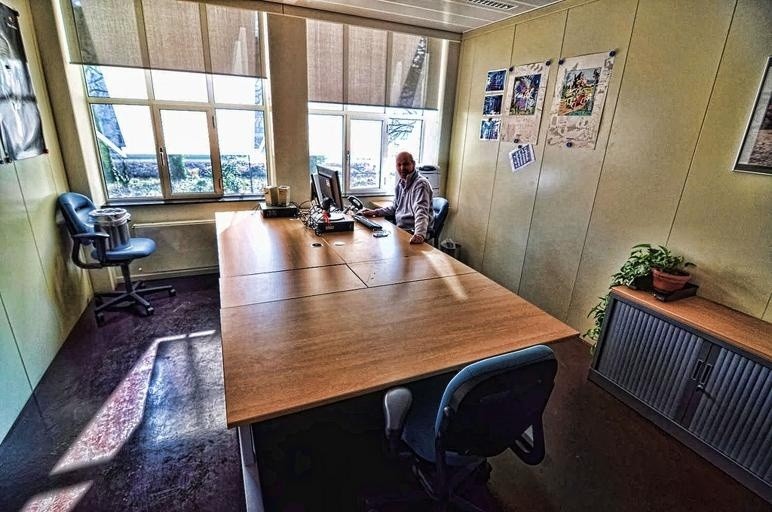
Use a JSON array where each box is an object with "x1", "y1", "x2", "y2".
[{"x1": 732, "y1": 56, "x2": 772, "y2": 177}]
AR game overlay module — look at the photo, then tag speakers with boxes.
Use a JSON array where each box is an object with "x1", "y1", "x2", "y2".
[
  {"x1": 264, "y1": 185, "x2": 277, "y2": 206},
  {"x1": 278, "y1": 185, "x2": 290, "y2": 206}
]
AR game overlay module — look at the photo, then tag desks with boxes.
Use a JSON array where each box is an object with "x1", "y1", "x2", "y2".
[{"x1": 215, "y1": 206, "x2": 583, "y2": 512}]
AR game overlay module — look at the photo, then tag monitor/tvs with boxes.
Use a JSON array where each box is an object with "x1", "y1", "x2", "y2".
[
  {"x1": 310, "y1": 173, "x2": 323, "y2": 208},
  {"x1": 315, "y1": 164, "x2": 345, "y2": 222}
]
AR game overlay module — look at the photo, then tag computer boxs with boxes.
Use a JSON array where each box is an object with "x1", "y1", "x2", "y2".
[
  {"x1": 259, "y1": 202, "x2": 297, "y2": 219},
  {"x1": 310, "y1": 211, "x2": 356, "y2": 234}
]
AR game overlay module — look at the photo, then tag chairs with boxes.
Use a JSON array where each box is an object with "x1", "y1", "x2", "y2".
[
  {"x1": 425, "y1": 196, "x2": 450, "y2": 247},
  {"x1": 58, "y1": 191, "x2": 176, "y2": 327}
]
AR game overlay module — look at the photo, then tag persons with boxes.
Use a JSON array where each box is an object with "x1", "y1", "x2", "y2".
[{"x1": 356, "y1": 150, "x2": 436, "y2": 245}]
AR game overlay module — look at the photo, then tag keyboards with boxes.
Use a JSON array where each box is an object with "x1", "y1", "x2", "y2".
[{"x1": 356, "y1": 214, "x2": 384, "y2": 230}]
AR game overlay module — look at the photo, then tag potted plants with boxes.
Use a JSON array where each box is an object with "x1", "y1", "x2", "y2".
[{"x1": 581, "y1": 241, "x2": 699, "y2": 359}]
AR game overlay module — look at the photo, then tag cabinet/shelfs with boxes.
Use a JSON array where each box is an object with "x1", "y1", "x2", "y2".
[{"x1": 393, "y1": 166, "x2": 441, "y2": 198}]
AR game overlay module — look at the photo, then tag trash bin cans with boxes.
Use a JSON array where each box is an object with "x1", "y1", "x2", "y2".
[{"x1": 441, "y1": 241, "x2": 464, "y2": 263}]
